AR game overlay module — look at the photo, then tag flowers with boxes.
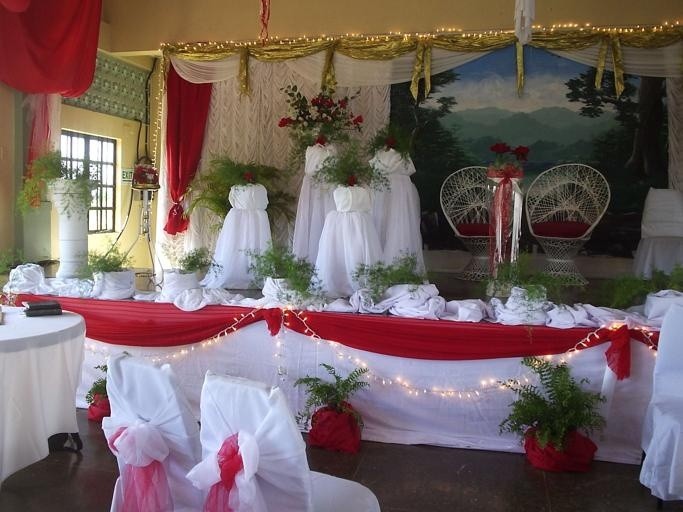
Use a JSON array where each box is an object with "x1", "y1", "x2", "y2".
[
  {"x1": 134, "y1": 157, "x2": 159, "y2": 183},
  {"x1": 204, "y1": 84, "x2": 416, "y2": 192},
  {"x1": 486, "y1": 139, "x2": 530, "y2": 177}
]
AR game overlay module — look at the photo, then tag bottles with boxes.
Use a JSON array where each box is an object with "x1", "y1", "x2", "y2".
[{"x1": 524, "y1": 238, "x2": 529, "y2": 252}]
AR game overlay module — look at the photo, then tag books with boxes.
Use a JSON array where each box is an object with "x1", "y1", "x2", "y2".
[{"x1": 22, "y1": 301, "x2": 63, "y2": 317}]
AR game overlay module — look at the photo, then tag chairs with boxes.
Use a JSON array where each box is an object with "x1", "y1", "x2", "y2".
[
  {"x1": 101, "y1": 351, "x2": 202, "y2": 512},
  {"x1": 184, "y1": 369, "x2": 380, "y2": 512},
  {"x1": 439, "y1": 164, "x2": 517, "y2": 282},
  {"x1": 632, "y1": 185, "x2": 683, "y2": 280},
  {"x1": 524, "y1": 162, "x2": 613, "y2": 287},
  {"x1": 638, "y1": 304, "x2": 683, "y2": 512}
]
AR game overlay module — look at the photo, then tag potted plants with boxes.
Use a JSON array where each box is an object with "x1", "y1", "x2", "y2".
[
  {"x1": 155, "y1": 247, "x2": 225, "y2": 304},
  {"x1": 473, "y1": 252, "x2": 577, "y2": 326},
  {"x1": 84, "y1": 351, "x2": 128, "y2": 421},
  {"x1": 15, "y1": 150, "x2": 103, "y2": 296},
  {"x1": 237, "y1": 238, "x2": 328, "y2": 310},
  {"x1": 0, "y1": 242, "x2": 53, "y2": 293},
  {"x1": 595, "y1": 261, "x2": 683, "y2": 320},
  {"x1": 348, "y1": 244, "x2": 438, "y2": 319},
  {"x1": 74, "y1": 237, "x2": 136, "y2": 301},
  {"x1": 288, "y1": 361, "x2": 371, "y2": 454},
  {"x1": 497, "y1": 353, "x2": 608, "y2": 474}
]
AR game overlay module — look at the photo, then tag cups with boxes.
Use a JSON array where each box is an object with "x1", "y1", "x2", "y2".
[{"x1": 532, "y1": 244, "x2": 538, "y2": 253}]
